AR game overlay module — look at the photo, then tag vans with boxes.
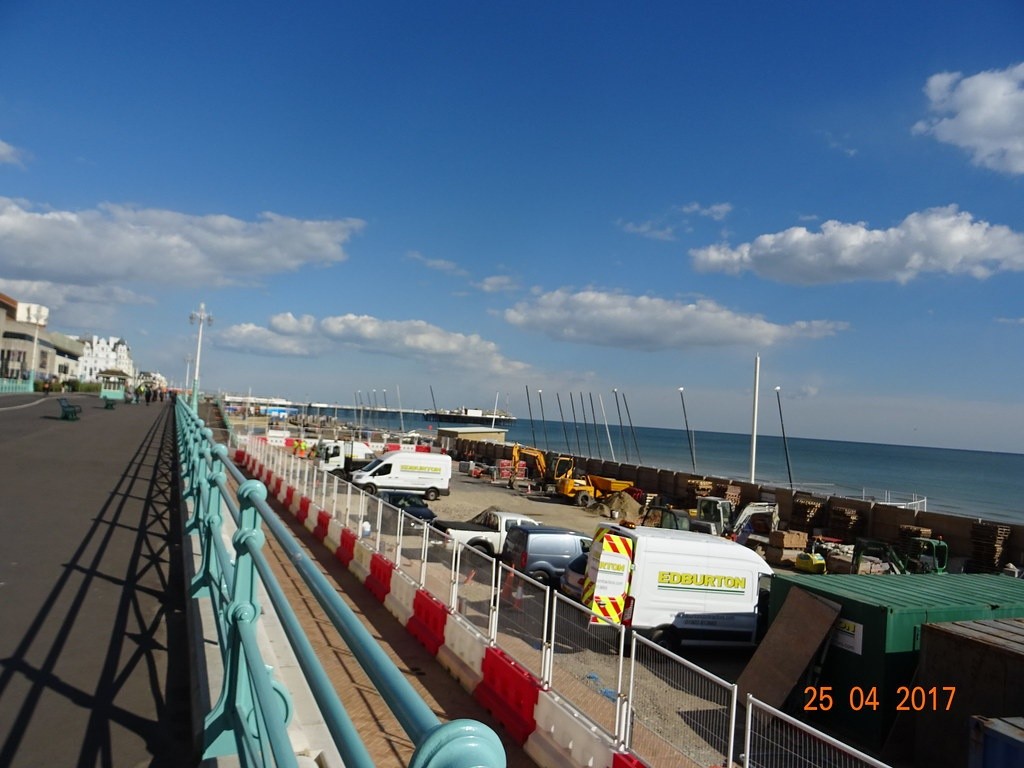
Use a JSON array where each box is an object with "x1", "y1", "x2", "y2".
[
  {"x1": 580, "y1": 521, "x2": 777, "y2": 658},
  {"x1": 505, "y1": 524, "x2": 593, "y2": 592},
  {"x1": 349, "y1": 451, "x2": 453, "y2": 502}
]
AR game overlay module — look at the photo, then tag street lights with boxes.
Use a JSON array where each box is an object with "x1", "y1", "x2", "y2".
[
  {"x1": 613, "y1": 388, "x2": 630, "y2": 464},
  {"x1": 189, "y1": 301, "x2": 212, "y2": 420},
  {"x1": 498, "y1": 563, "x2": 514, "y2": 604},
  {"x1": 511, "y1": 578, "x2": 533, "y2": 614},
  {"x1": 28, "y1": 306, "x2": 45, "y2": 393},
  {"x1": 537, "y1": 389, "x2": 549, "y2": 450},
  {"x1": 775, "y1": 386, "x2": 793, "y2": 488},
  {"x1": 382, "y1": 388, "x2": 391, "y2": 432},
  {"x1": 677, "y1": 386, "x2": 696, "y2": 473}
]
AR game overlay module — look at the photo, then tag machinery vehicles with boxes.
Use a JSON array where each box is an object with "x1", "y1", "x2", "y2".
[
  {"x1": 845, "y1": 534, "x2": 949, "y2": 577},
  {"x1": 642, "y1": 494, "x2": 782, "y2": 554},
  {"x1": 507, "y1": 440, "x2": 633, "y2": 508}
]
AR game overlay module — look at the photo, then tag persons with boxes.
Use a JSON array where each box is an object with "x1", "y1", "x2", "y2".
[
  {"x1": 124, "y1": 384, "x2": 171, "y2": 407},
  {"x1": 293, "y1": 439, "x2": 317, "y2": 459}
]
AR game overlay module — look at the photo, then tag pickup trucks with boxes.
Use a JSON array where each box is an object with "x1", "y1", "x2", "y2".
[{"x1": 432, "y1": 508, "x2": 541, "y2": 566}]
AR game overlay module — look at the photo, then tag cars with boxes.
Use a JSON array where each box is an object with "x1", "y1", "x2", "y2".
[
  {"x1": 559, "y1": 553, "x2": 602, "y2": 605},
  {"x1": 367, "y1": 493, "x2": 437, "y2": 532}
]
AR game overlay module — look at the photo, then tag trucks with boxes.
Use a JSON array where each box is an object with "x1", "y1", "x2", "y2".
[{"x1": 313, "y1": 439, "x2": 381, "y2": 482}]
